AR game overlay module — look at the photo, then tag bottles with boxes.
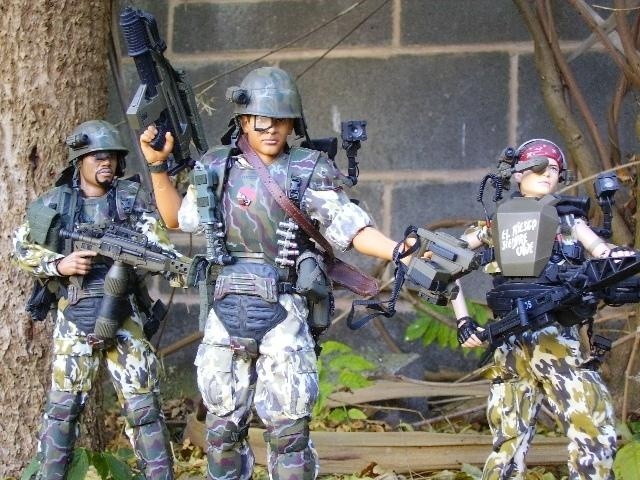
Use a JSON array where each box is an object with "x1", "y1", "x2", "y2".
[{"x1": 516, "y1": 140, "x2": 564, "y2": 171}]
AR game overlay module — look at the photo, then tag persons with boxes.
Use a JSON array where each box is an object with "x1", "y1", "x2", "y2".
[
  {"x1": 444, "y1": 138, "x2": 630, "y2": 480},
  {"x1": 10, "y1": 116, "x2": 183, "y2": 480},
  {"x1": 138, "y1": 69, "x2": 434, "y2": 480}
]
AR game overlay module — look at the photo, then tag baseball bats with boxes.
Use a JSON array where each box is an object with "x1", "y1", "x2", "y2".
[
  {"x1": 477, "y1": 255, "x2": 640, "y2": 368},
  {"x1": 405, "y1": 227, "x2": 481, "y2": 305},
  {"x1": 119, "y1": 4, "x2": 209, "y2": 175},
  {"x1": 57, "y1": 221, "x2": 210, "y2": 340}
]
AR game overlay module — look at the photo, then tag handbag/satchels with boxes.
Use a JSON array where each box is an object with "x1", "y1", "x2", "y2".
[{"x1": 148, "y1": 160, "x2": 168, "y2": 174}]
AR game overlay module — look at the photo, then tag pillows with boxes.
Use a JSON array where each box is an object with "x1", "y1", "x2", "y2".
[
  {"x1": 226, "y1": 67, "x2": 303, "y2": 119},
  {"x1": 67, "y1": 119, "x2": 129, "y2": 163}
]
